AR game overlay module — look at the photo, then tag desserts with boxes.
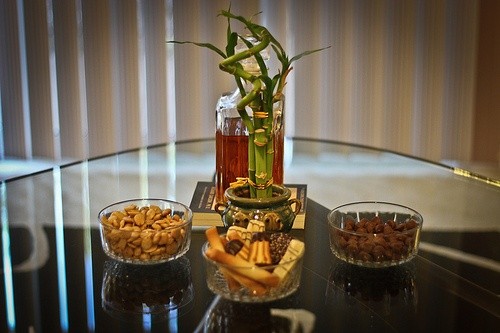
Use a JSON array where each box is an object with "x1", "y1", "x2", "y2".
[{"x1": 204, "y1": 219, "x2": 306, "y2": 295}]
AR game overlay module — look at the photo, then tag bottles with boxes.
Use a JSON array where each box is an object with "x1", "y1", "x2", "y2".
[{"x1": 215, "y1": 34, "x2": 285, "y2": 202}]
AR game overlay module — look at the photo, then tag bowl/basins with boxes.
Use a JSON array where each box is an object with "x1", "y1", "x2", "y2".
[
  {"x1": 327, "y1": 201, "x2": 423, "y2": 267},
  {"x1": 201, "y1": 232, "x2": 305, "y2": 303},
  {"x1": 98, "y1": 198, "x2": 192, "y2": 265}
]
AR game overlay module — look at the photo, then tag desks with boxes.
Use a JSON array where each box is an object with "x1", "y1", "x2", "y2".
[{"x1": 0, "y1": 134, "x2": 500, "y2": 332}]
